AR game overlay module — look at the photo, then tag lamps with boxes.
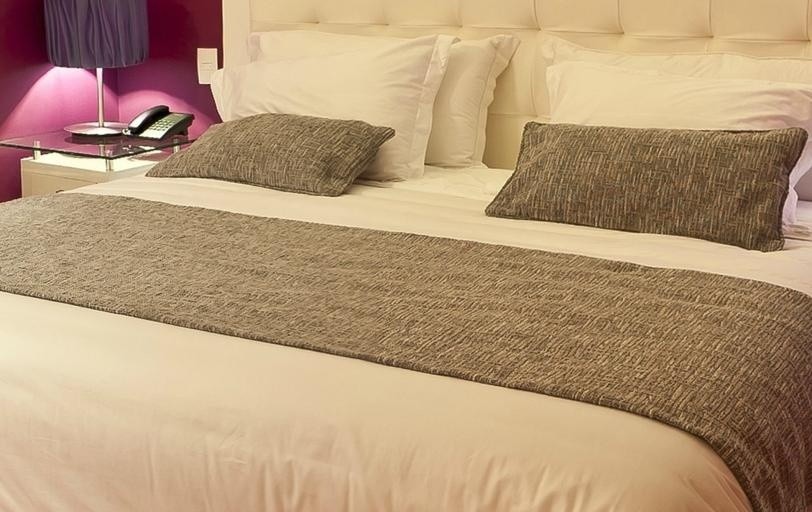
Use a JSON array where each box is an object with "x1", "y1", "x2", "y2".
[{"x1": 44, "y1": 0, "x2": 149, "y2": 135}]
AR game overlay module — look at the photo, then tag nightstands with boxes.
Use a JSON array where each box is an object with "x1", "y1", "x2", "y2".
[{"x1": 0, "y1": 134, "x2": 196, "y2": 198}]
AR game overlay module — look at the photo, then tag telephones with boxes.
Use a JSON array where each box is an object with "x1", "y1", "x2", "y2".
[{"x1": 121, "y1": 105, "x2": 195, "y2": 142}]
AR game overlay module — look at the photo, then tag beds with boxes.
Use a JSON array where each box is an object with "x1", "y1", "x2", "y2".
[{"x1": 0, "y1": 0, "x2": 812, "y2": 512}]
